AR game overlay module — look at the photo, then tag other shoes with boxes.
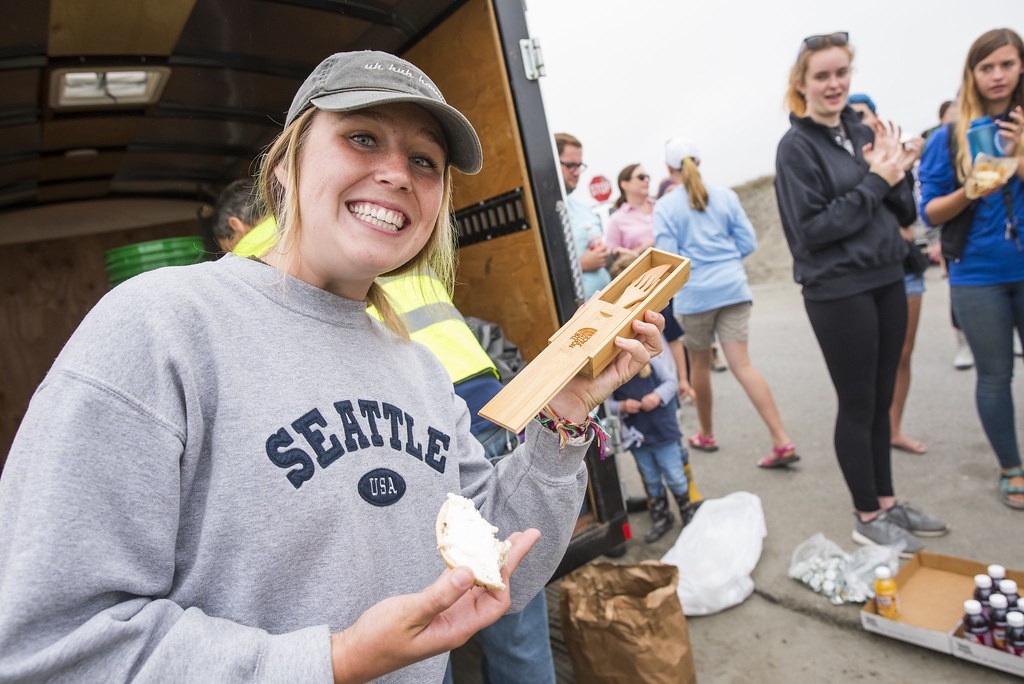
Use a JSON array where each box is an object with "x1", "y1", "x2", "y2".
[
  {"x1": 890, "y1": 430, "x2": 927, "y2": 454},
  {"x1": 953, "y1": 333, "x2": 975, "y2": 370},
  {"x1": 643, "y1": 514, "x2": 677, "y2": 542}
]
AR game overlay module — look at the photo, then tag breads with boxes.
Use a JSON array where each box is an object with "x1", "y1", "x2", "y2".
[{"x1": 436, "y1": 493, "x2": 508, "y2": 591}]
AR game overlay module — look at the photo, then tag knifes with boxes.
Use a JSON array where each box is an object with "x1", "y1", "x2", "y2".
[{"x1": 625, "y1": 263, "x2": 673, "y2": 309}]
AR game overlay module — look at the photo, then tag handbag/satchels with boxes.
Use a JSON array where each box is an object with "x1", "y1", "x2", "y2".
[{"x1": 560, "y1": 558, "x2": 697, "y2": 684}]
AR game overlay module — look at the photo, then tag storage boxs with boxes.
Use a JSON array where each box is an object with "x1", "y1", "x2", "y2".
[
  {"x1": 859, "y1": 552, "x2": 1024, "y2": 678},
  {"x1": 478, "y1": 247, "x2": 691, "y2": 434}
]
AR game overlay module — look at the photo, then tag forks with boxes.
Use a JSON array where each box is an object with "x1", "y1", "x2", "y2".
[{"x1": 614, "y1": 272, "x2": 661, "y2": 309}]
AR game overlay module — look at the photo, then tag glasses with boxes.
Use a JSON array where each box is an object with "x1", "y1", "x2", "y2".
[
  {"x1": 797, "y1": 31, "x2": 849, "y2": 64},
  {"x1": 559, "y1": 160, "x2": 588, "y2": 172},
  {"x1": 629, "y1": 174, "x2": 651, "y2": 181}
]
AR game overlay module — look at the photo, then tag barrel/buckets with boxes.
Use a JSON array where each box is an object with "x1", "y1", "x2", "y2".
[{"x1": 103, "y1": 234, "x2": 210, "y2": 290}]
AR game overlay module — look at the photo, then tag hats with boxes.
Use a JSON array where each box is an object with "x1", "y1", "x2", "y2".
[
  {"x1": 285, "y1": 49, "x2": 484, "y2": 177},
  {"x1": 664, "y1": 138, "x2": 699, "y2": 168}
]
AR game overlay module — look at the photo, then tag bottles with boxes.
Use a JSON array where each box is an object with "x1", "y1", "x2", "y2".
[
  {"x1": 583, "y1": 225, "x2": 604, "y2": 250},
  {"x1": 967, "y1": 115, "x2": 1004, "y2": 165},
  {"x1": 961, "y1": 565, "x2": 1024, "y2": 658},
  {"x1": 873, "y1": 566, "x2": 901, "y2": 621}
]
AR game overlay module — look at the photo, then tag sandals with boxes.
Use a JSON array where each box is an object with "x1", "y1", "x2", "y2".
[
  {"x1": 998, "y1": 466, "x2": 1024, "y2": 508},
  {"x1": 688, "y1": 431, "x2": 719, "y2": 452},
  {"x1": 757, "y1": 444, "x2": 802, "y2": 466}
]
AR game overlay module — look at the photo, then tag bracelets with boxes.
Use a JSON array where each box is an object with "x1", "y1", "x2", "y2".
[{"x1": 537, "y1": 405, "x2": 611, "y2": 461}]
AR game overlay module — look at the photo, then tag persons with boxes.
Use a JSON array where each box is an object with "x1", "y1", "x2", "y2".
[
  {"x1": 0, "y1": 51, "x2": 664, "y2": 684},
  {"x1": 772, "y1": 31, "x2": 950, "y2": 558},
  {"x1": 555, "y1": 132, "x2": 802, "y2": 542},
  {"x1": 917, "y1": 28, "x2": 1024, "y2": 509},
  {"x1": 208, "y1": 178, "x2": 557, "y2": 684}
]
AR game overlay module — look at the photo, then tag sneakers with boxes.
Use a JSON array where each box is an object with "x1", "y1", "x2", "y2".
[{"x1": 851, "y1": 500, "x2": 951, "y2": 557}]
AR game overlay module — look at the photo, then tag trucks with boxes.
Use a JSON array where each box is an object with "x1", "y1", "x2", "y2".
[{"x1": 3, "y1": 1, "x2": 634, "y2": 586}]
